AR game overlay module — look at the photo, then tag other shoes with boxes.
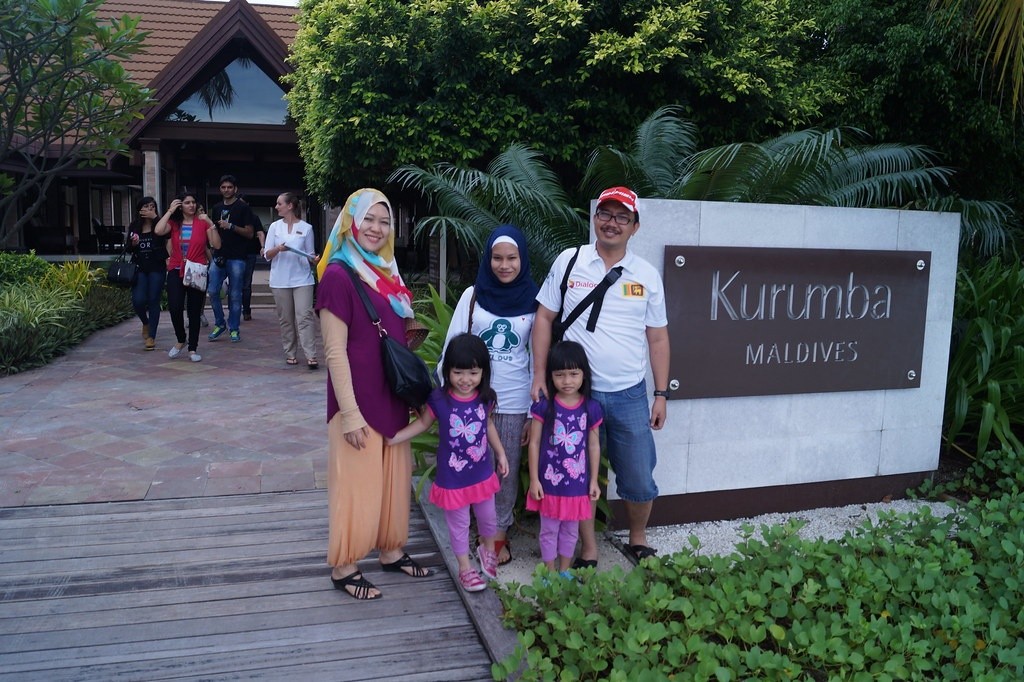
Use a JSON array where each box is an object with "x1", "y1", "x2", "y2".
[
  {"x1": 143, "y1": 338, "x2": 156, "y2": 351},
  {"x1": 142, "y1": 323, "x2": 149, "y2": 340},
  {"x1": 187, "y1": 354, "x2": 201, "y2": 362},
  {"x1": 168, "y1": 339, "x2": 188, "y2": 359}
]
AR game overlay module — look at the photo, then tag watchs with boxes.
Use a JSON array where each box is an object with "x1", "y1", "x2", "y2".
[
  {"x1": 653, "y1": 391, "x2": 670, "y2": 400},
  {"x1": 229, "y1": 224, "x2": 235, "y2": 230}
]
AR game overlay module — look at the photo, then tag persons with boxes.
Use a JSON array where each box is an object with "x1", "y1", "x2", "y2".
[
  {"x1": 124, "y1": 174, "x2": 265, "y2": 363},
  {"x1": 263, "y1": 192, "x2": 320, "y2": 369},
  {"x1": 386, "y1": 333, "x2": 510, "y2": 592},
  {"x1": 317, "y1": 189, "x2": 435, "y2": 599},
  {"x1": 433, "y1": 225, "x2": 541, "y2": 567},
  {"x1": 526, "y1": 342, "x2": 603, "y2": 581},
  {"x1": 532, "y1": 187, "x2": 671, "y2": 584}
]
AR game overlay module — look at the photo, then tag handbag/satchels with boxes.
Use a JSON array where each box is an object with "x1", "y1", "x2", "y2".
[
  {"x1": 183, "y1": 259, "x2": 208, "y2": 292},
  {"x1": 550, "y1": 308, "x2": 564, "y2": 349},
  {"x1": 379, "y1": 333, "x2": 432, "y2": 407},
  {"x1": 107, "y1": 245, "x2": 139, "y2": 289}
]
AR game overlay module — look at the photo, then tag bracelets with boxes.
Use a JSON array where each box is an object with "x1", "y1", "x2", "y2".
[
  {"x1": 210, "y1": 224, "x2": 216, "y2": 229},
  {"x1": 168, "y1": 209, "x2": 174, "y2": 214},
  {"x1": 262, "y1": 246, "x2": 266, "y2": 249}
]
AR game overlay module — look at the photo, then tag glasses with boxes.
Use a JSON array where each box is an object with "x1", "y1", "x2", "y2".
[{"x1": 596, "y1": 211, "x2": 635, "y2": 225}]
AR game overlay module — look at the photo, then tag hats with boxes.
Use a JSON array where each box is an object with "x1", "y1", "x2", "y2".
[{"x1": 595, "y1": 186, "x2": 641, "y2": 212}]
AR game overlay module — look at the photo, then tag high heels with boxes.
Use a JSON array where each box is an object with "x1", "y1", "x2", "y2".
[
  {"x1": 331, "y1": 570, "x2": 383, "y2": 600},
  {"x1": 381, "y1": 552, "x2": 430, "y2": 577}
]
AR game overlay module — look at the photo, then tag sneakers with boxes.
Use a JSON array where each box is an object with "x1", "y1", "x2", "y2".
[
  {"x1": 244, "y1": 313, "x2": 251, "y2": 321},
  {"x1": 541, "y1": 570, "x2": 583, "y2": 593},
  {"x1": 229, "y1": 328, "x2": 241, "y2": 343},
  {"x1": 477, "y1": 543, "x2": 498, "y2": 580},
  {"x1": 185, "y1": 314, "x2": 209, "y2": 328},
  {"x1": 458, "y1": 568, "x2": 487, "y2": 592},
  {"x1": 207, "y1": 322, "x2": 228, "y2": 342}
]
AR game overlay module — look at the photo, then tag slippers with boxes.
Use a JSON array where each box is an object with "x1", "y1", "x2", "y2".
[
  {"x1": 623, "y1": 543, "x2": 657, "y2": 564},
  {"x1": 286, "y1": 358, "x2": 318, "y2": 368},
  {"x1": 475, "y1": 533, "x2": 512, "y2": 565},
  {"x1": 572, "y1": 555, "x2": 597, "y2": 585}
]
